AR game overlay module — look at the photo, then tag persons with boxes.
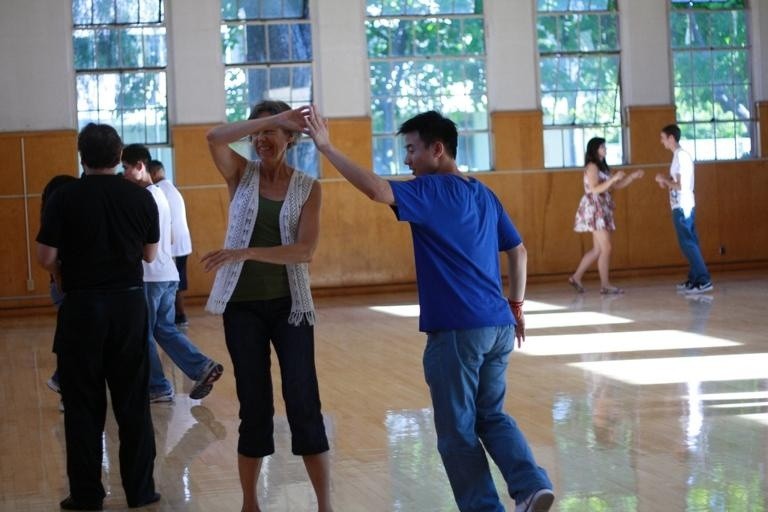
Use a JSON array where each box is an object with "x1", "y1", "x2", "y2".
[
  {"x1": 568, "y1": 138, "x2": 644, "y2": 295},
  {"x1": 304, "y1": 102, "x2": 555, "y2": 512},
  {"x1": 121, "y1": 143, "x2": 224, "y2": 405},
  {"x1": 38, "y1": 123, "x2": 162, "y2": 512},
  {"x1": 655, "y1": 125, "x2": 714, "y2": 295},
  {"x1": 207, "y1": 100, "x2": 334, "y2": 511},
  {"x1": 42, "y1": 174, "x2": 82, "y2": 412},
  {"x1": 147, "y1": 159, "x2": 193, "y2": 331}
]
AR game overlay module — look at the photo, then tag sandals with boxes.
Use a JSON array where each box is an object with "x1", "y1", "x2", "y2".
[
  {"x1": 568, "y1": 277, "x2": 587, "y2": 292},
  {"x1": 599, "y1": 286, "x2": 624, "y2": 295}
]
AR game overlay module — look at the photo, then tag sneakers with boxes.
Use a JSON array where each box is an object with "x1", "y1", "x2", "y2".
[
  {"x1": 58, "y1": 398, "x2": 64, "y2": 412},
  {"x1": 677, "y1": 280, "x2": 694, "y2": 289},
  {"x1": 189, "y1": 361, "x2": 224, "y2": 400},
  {"x1": 145, "y1": 389, "x2": 175, "y2": 403},
  {"x1": 516, "y1": 486, "x2": 555, "y2": 512},
  {"x1": 684, "y1": 279, "x2": 714, "y2": 294},
  {"x1": 46, "y1": 378, "x2": 61, "y2": 394},
  {"x1": 174, "y1": 313, "x2": 188, "y2": 326}
]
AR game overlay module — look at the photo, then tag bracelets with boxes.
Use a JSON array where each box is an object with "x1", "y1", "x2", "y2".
[{"x1": 507, "y1": 297, "x2": 524, "y2": 308}]
